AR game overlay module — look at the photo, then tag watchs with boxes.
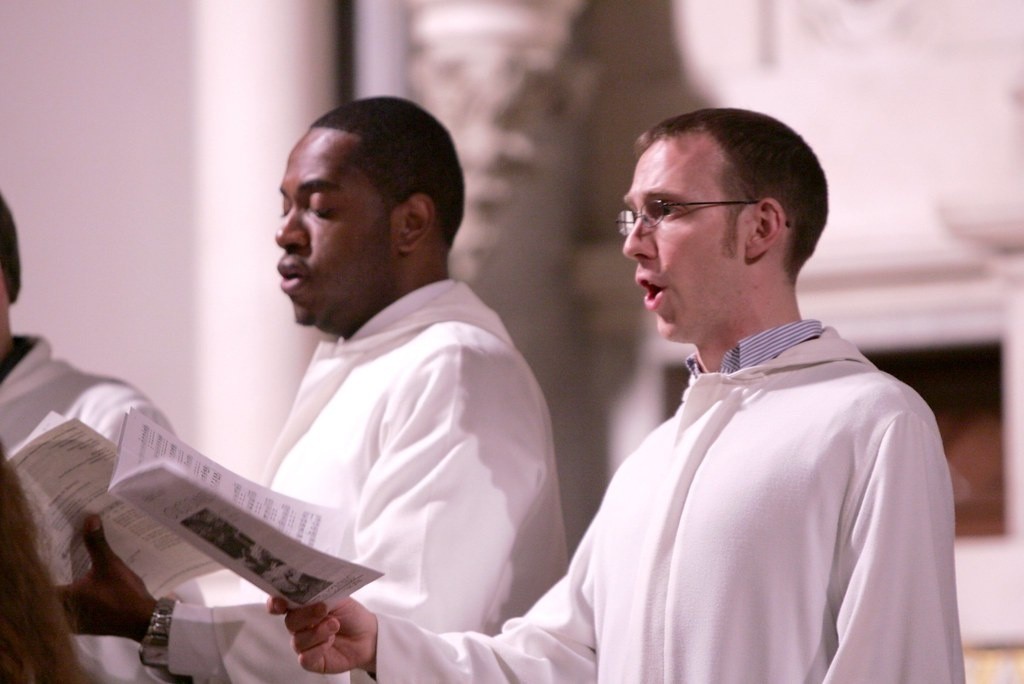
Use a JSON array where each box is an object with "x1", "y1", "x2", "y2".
[{"x1": 137, "y1": 596, "x2": 176, "y2": 668}]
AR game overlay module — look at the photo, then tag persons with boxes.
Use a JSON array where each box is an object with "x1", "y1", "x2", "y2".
[
  {"x1": 51, "y1": 96, "x2": 565, "y2": 684},
  {"x1": 0, "y1": 195, "x2": 208, "y2": 684},
  {"x1": 266, "y1": 108, "x2": 967, "y2": 684}
]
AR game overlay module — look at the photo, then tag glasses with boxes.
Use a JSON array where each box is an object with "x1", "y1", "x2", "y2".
[{"x1": 614, "y1": 200, "x2": 791, "y2": 237}]
